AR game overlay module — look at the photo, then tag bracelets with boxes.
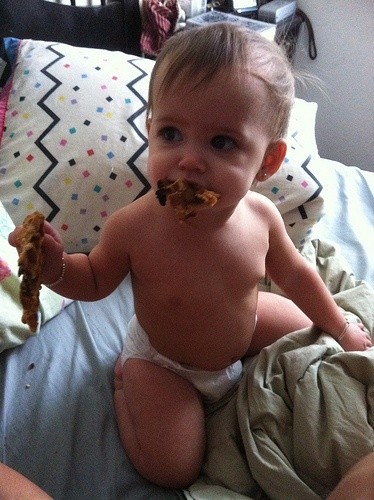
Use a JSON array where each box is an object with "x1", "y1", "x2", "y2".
[
  {"x1": 44, "y1": 256, "x2": 66, "y2": 287},
  {"x1": 335, "y1": 319, "x2": 351, "y2": 343}
]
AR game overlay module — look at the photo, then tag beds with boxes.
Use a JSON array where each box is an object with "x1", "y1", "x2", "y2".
[{"x1": 0, "y1": 156, "x2": 374, "y2": 500}]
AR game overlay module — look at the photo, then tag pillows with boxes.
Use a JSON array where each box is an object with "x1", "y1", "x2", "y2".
[{"x1": 0, "y1": 34, "x2": 325, "y2": 266}]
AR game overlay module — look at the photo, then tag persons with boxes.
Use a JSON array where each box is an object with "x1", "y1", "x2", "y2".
[{"x1": 8, "y1": 21, "x2": 373, "y2": 488}]
